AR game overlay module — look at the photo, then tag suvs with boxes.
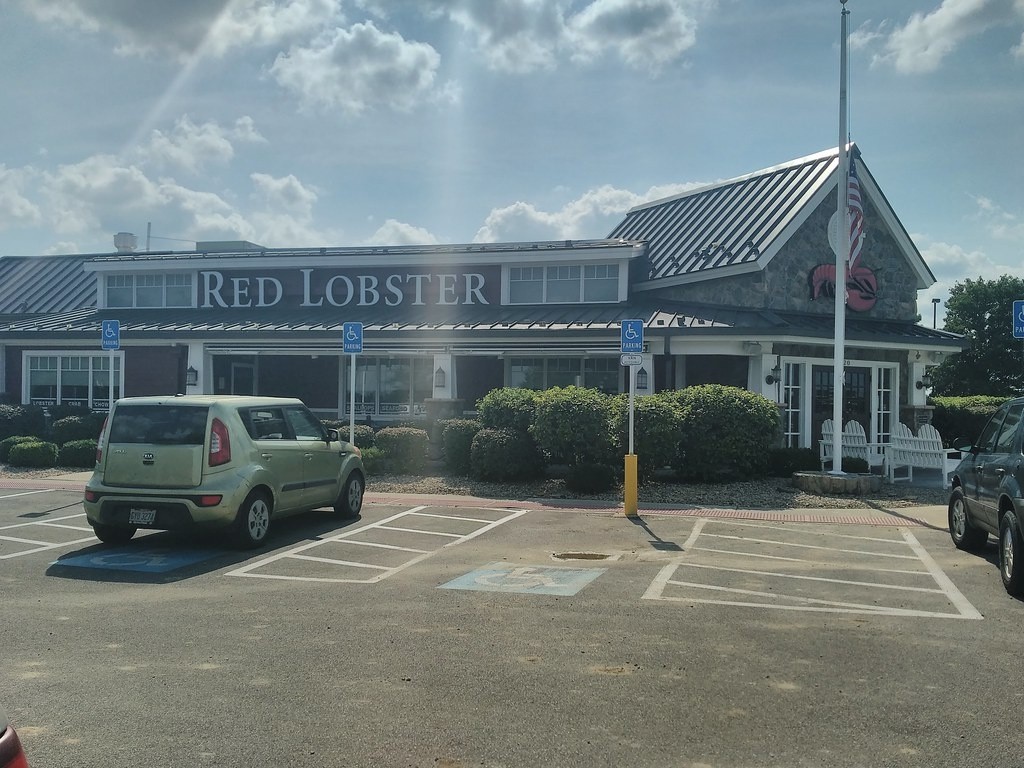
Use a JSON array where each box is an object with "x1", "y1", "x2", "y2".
[
  {"x1": 948, "y1": 396, "x2": 1024, "y2": 599},
  {"x1": 83, "y1": 395, "x2": 367, "y2": 550}
]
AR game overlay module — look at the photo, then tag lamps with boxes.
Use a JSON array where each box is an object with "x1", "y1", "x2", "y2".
[
  {"x1": 766, "y1": 364, "x2": 782, "y2": 385},
  {"x1": 186, "y1": 365, "x2": 197, "y2": 385},
  {"x1": 636, "y1": 367, "x2": 647, "y2": 390},
  {"x1": 916, "y1": 370, "x2": 931, "y2": 390},
  {"x1": 435, "y1": 366, "x2": 445, "y2": 388}
]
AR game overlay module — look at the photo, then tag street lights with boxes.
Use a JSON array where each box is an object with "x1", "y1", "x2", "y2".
[{"x1": 932, "y1": 298, "x2": 941, "y2": 329}]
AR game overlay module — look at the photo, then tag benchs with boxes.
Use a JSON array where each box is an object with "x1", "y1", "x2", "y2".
[
  {"x1": 887, "y1": 423, "x2": 967, "y2": 490},
  {"x1": 818, "y1": 419, "x2": 890, "y2": 478}
]
